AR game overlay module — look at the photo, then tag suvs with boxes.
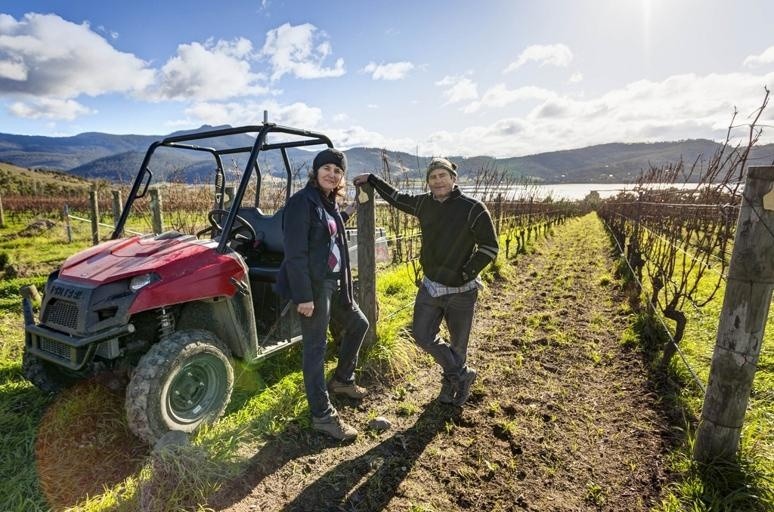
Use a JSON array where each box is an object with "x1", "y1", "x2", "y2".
[{"x1": 21, "y1": 108, "x2": 389, "y2": 452}]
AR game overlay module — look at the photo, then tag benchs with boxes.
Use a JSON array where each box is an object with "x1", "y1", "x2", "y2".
[{"x1": 210, "y1": 205, "x2": 289, "y2": 283}]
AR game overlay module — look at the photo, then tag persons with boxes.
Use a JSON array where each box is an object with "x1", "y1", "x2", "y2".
[
  {"x1": 350, "y1": 159, "x2": 499, "y2": 409},
  {"x1": 276, "y1": 146, "x2": 368, "y2": 440}
]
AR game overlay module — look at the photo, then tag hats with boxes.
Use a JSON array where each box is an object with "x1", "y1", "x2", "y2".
[
  {"x1": 314, "y1": 148, "x2": 347, "y2": 172},
  {"x1": 427, "y1": 157, "x2": 458, "y2": 183}
]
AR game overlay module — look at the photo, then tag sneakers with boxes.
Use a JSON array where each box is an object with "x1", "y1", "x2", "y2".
[
  {"x1": 440, "y1": 366, "x2": 477, "y2": 405},
  {"x1": 311, "y1": 409, "x2": 358, "y2": 443},
  {"x1": 327, "y1": 378, "x2": 368, "y2": 399}
]
